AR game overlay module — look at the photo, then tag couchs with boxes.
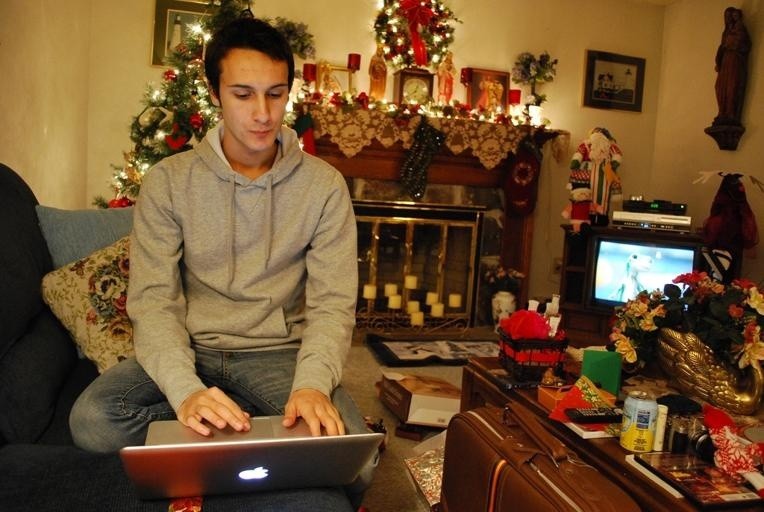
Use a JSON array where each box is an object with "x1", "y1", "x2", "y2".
[{"x1": 0, "y1": 161, "x2": 350, "y2": 512}]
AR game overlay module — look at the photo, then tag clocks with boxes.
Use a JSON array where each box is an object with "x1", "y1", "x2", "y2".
[{"x1": 394, "y1": 65, "x2": 435, "y2": 106}]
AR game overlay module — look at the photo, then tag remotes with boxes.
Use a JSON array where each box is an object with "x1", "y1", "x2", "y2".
[{"x1": 564, "y1": 406, "x2": 622, "y2": 424}]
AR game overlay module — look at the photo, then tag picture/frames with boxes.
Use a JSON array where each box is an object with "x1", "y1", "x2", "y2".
[
  {"x1": 319, "y1": 66, "x2": 353, "y2": 96},
  {"x1": 466, "y1": 66, "x2": 511, "y2": 113},
  {"x1": 151, "y1": 0, "x2": 221, "y2": 67},
  {"x1": 585, "y1": 51, "x2": 647, "y2": 113}
]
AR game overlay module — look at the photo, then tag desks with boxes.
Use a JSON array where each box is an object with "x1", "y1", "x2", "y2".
[{"x1": 459, "y1": 354, "x2": 764, "y2": 510}]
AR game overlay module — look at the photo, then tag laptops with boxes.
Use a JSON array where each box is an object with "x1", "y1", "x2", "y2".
[{"x1": 120, "y1": 410, "x2": 385, "y2": 502}]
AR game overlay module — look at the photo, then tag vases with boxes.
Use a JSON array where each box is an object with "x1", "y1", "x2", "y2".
[
  {"x1": 492, "y1": 290, "x2": 517, "y2": 333},
  {"x1": 528, "y1": 104, "x2": 544, "y2": 126}
]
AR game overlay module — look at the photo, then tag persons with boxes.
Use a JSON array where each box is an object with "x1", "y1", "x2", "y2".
[
  {"x1": 68, "y1": 16, "x2": 381, "y2": 512},
  {"x1": 0, "y1": 163, "x2": 59, "y2": 445},
  {"x1": 714, "y1": 7, "x2": 752, "y2": 123}
]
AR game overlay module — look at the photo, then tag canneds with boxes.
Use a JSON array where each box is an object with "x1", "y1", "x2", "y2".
[{"x1": 619, "y1": 390, "x2": 658, "y2": 454}]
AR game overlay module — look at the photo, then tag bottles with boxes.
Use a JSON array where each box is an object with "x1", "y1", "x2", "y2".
[{"x1": 650, "y1": 403, "x2": 706, "y2": 457}]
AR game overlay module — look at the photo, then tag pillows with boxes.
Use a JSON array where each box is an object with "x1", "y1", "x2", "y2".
[
  {"x1": 41, "y1": 234, "x2": 133, "y2": 374},
  {"x1": 33, "y1": 199, "x2": 134, "y2": 269}
]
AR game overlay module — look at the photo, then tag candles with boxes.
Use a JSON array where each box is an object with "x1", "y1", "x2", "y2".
[
  {"x1": 348, "y1": 53, "x2": 361, "y2": 70},
  {"x1": 509, "y1": 90, "x2": 521, "y2": 105},
  {"x1": 303, "y1": 64, "x2": 316, "y2": 81},
  {"x1": 362, "y1": 274, "x2": 462, "y2": 326}
]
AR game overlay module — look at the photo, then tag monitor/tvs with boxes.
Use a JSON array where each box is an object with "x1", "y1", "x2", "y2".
[{"x1": 587, "y1": 235, "x2": 697, "y2": 311}]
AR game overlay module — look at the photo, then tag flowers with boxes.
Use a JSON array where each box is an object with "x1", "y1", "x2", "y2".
[
  {"x1": 267, "y1": 18, "x2": 317, "y2": 63},
  {"x1": 512, "y1": 51, "x2": 558, "y2": 104},
  {"x1": 608, "y1": 269, "x2": 764, "y2": 374},
  {"x1": 483, "y1": 266, "x2": 527, "y2": 292}
]
